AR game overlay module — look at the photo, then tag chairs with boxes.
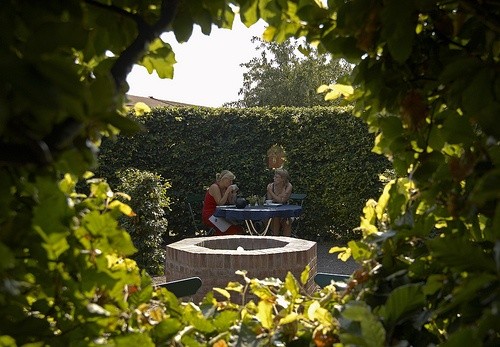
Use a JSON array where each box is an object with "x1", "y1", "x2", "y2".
[
  {"x1": 288, "y1": 193, "x2": 307, "y2": 239},
  {"x1": 187, "y1": 192, "x2": 215, "y2": 236},
  {"x1": 313, "y1": 273, "x2": 352, "y2": 296},
  {"x1": 154, "y1": 276, "x2": 203, "y2": 302}
]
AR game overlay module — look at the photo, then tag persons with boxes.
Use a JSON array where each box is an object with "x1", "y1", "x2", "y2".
[
  {"x1": 202, "y1": 170, "x2": 244, "y2": 236},
  {"x1": 267, "y1": 168, "x2": 293, "y2": 237}
]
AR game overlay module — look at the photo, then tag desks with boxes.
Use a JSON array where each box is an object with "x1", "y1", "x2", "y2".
[{"x1": 214, "y1": 202, "x2": 302, "y2": 235}]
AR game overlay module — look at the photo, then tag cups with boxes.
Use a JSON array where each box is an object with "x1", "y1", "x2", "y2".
[
  {"x1": 231, "y1": 185, "x2": 236, "y2": 190},
  {"x1": 266, "y1": 200, "x2": 272, "y2": 204}
]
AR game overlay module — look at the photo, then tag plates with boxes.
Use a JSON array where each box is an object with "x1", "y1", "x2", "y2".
[
  {"x1": 216, "y1": 204, "x2": 236, "y2": 208},
  {"x1": 264, "y1": 204, "x2": 282, "y2": 206}
]
